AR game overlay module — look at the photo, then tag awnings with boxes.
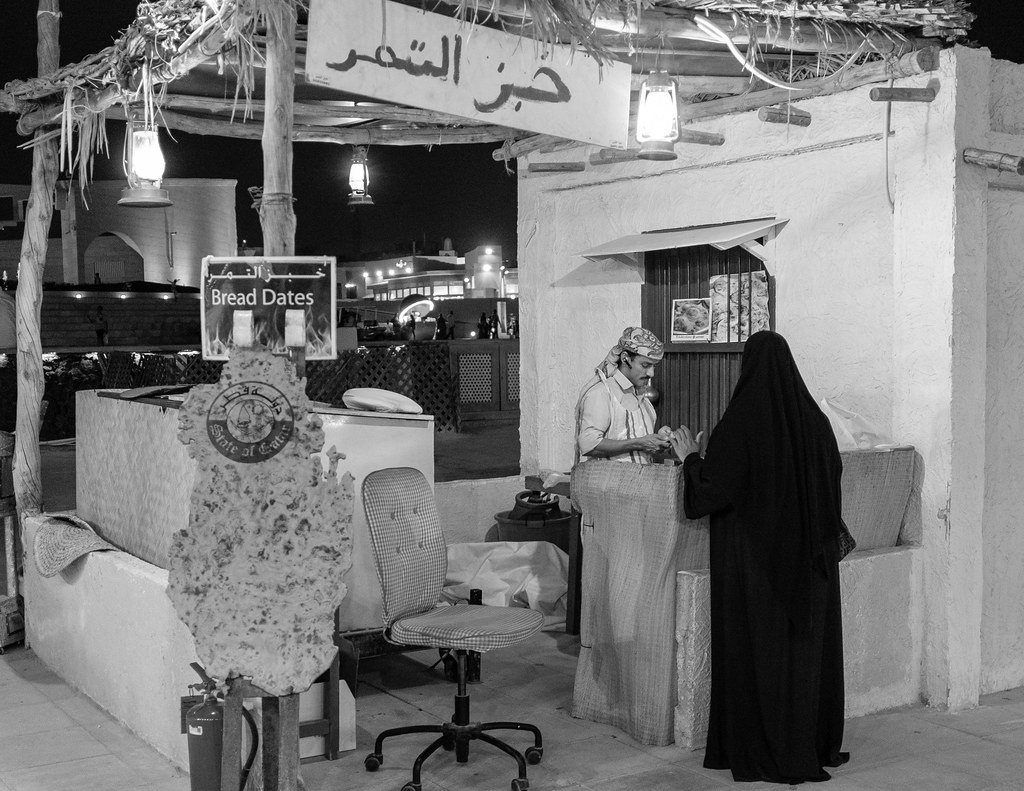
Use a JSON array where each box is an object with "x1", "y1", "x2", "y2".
[{"x1": 569, "y1": 220, "x2": 794, "y2": 268}]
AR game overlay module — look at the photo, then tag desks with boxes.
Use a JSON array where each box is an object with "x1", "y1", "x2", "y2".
[
  {"x1": 73, "y1": 391, "x2": 441, "y2": 632},
  {"x1": 572, "y1": 446, "x2": 919, "y2": 744}
]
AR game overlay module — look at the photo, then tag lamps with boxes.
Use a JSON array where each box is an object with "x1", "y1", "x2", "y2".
[
  {"x1": 347, "y1": 149, "x2": 373, "y2": 207},
  {"x1": 635, "y1": 46, "x2": 680, "y2": 162},
  {"x1": 117, "y1": 109, "x2": 176, "y2": 207}
]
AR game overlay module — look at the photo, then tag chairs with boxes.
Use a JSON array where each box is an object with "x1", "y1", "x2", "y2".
[{"x1": 362, "y1": 468, "x2": 546, "y2": 791}]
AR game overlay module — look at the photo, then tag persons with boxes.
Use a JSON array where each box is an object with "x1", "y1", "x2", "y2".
[
  {"x1": 87, "y1": 305, "x2": 109, "y2": 346},
  {"x1": 437, "y1": 313, "x2": 446, "y2": 340},
  {"x1": 668, "y1": 331, "x2": 851, "y2": 785},
  {"x1": 0, "y1": 286, "x2": 21, "y2": 518},
  {"x1": 390, "y1": 312, "x2": 399, "y2": 323},
  {"x1": 447, "y1": 310, "x2": 455, "y2": 340},
  {"x1": 410, "y1": 315, "x2": 416, "y2": 340},
  {"x1": 489, "y1": 309, "x2": 502, "y2": 339},
  {"x1": 509, "y1": 313, "x2": 518, "y2": 337},
  {"x1": 478, "y1": 312, "x2": 490, "y2": 339},
  {"x1": 385, "y1": 320, "x2": 395, "y2": 336},
  {"x1": 573, "y1": 327, "x2": 675, "y2": 464},
  {"x1": 488, "y1": 316, "x2": 491, "y2": 324},
  {"x1": 95, "y1": 272, "x2": 101, "y2": 284}
]
